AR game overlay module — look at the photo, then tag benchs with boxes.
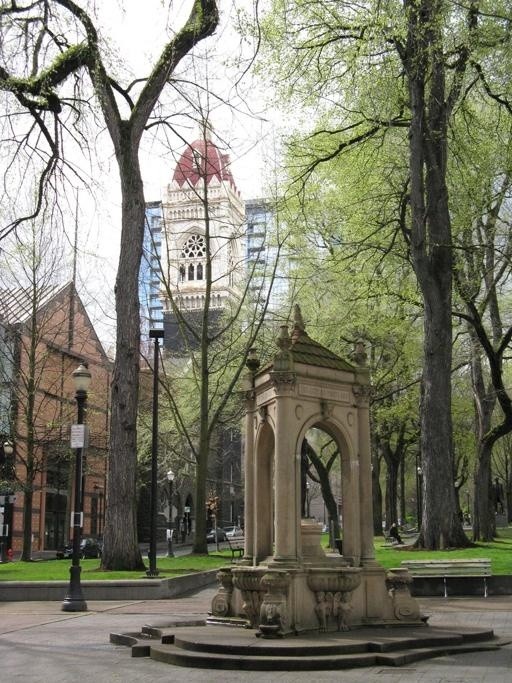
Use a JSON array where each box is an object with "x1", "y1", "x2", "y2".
[
  {"x1": 383, "y1": 531, "x2": 396, "y2": 543},
  {"x1": 227, "y1": 536, "x2": 244, "y2": 559},
  {"x1": 401, "y1": 558, "x2": 493, "y2": 597}
]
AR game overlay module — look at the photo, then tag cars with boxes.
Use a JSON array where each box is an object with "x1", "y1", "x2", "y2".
[
  {"x1": 206, "y1": 526, "x2": 244, "y2": 543},
  {"x1": 56, "y1": 536, "x2": 101, "y2": 559}
]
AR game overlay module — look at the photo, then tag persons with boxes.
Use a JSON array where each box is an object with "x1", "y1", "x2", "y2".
[{"x1": 389, "y1": 522, "x2": 405, "y2": 544}]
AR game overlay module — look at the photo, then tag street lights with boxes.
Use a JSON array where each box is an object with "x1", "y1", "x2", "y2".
[
  {"x1": 417, "y1": 466, "x2": 472, "y2": 531},
  {"x1": 60, "y1": 363, "x2": 92, "y2": 612},
  {"x1": 0, "y1": 440, "x2": 14, "y2": 563},
  {"x1": 306, "y1": 481, "x2": 311, "y2": 518},
  {"x1": 166, "y1": 469, "x2": 175, "y2": 558}
]
